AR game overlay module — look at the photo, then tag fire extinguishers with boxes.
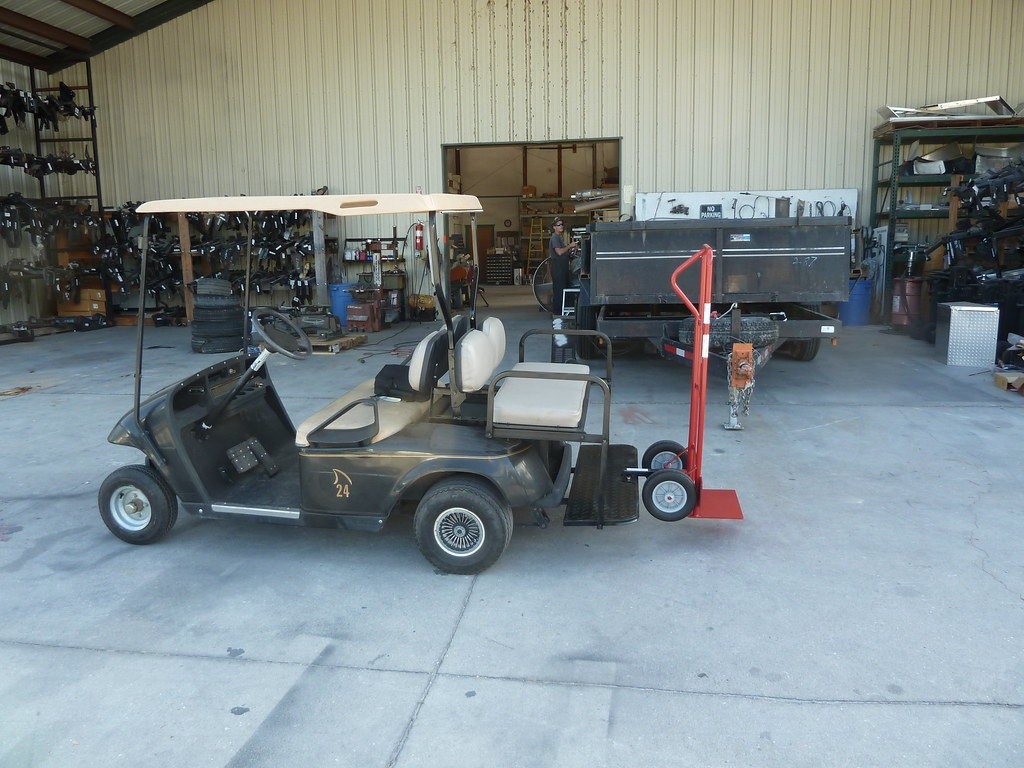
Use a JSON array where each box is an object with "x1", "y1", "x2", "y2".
[{"x1": 416, "y1": 220, "x2": 426, "y2": 250}]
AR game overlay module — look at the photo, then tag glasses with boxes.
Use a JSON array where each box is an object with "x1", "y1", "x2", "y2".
[{"x1": 553, "y1": 223, "x2": 564, "y2": 226}]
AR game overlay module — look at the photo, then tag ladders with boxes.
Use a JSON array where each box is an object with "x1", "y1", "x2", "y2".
[{"x1": 524, "y1": 218, "x2": 545, "y2": 286}]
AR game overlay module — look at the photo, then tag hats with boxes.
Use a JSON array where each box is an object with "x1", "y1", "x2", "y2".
[{"x1": 552, "y1": 217, "x2": 564, "y2": 225}]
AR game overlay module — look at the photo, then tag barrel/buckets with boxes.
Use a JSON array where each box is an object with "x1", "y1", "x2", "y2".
[
  {"x1": 891, "y1": 277, "x2": 921, "y2": 326},
  {"x1": 837, "y1": 280, "x2": 874, "y2": 326},
  {"x1": 328, "y1": 283, "x2": 355, "y2": 326}
]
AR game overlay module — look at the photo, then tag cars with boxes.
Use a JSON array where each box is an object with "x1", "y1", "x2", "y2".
[{"x1": 95, "y1": 193, "x2": 641, "y2": 574}]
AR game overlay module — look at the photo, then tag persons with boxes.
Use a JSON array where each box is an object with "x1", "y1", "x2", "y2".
[{"x1": 548, "y1": 215, "x2": 580, "y2": 318}]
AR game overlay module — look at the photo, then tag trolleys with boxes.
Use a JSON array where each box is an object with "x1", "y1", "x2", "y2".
[{"x1": 640, "y1": 243, "x2": 745, "y2": 521}]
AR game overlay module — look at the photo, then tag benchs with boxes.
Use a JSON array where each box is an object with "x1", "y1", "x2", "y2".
[
  {"x1": 455, "y1": 314, "x2": 592, "y2": 438},
  {"x1": 294, "y1": 305, "x2": 467, "y2": 452}
]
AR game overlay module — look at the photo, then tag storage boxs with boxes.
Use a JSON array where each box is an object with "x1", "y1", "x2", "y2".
[{"x1": 56, "y1": 288, "x2": 107, "y2": 317}]
